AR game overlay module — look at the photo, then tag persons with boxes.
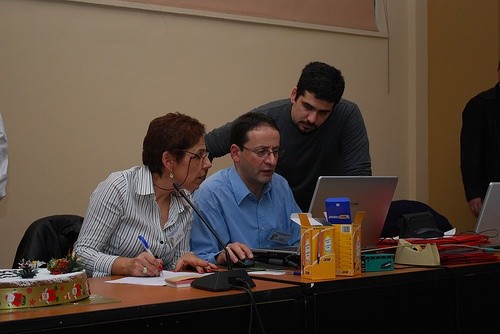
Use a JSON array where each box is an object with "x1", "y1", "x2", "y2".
[
  {"x1": 460, "y1": 61, "x2": 500, "y2": 210},
  {"x1": 205, "y1": 61, "x2": 373, "y2": 213},
  {"x1": 72, "y1": 112, "x2": 218, "y2": 277},
  {"x1": 189, "y1": 113, "x2": 304, "y2": 266}
]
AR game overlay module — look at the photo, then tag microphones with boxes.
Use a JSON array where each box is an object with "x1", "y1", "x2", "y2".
[{"x1": 173, "y1": 181, "x2": 256, "y2": 291}]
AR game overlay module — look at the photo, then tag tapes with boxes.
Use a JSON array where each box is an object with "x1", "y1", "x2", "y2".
[{"x1": 411, "y1": 245, "x2": 422, "y2": 251}]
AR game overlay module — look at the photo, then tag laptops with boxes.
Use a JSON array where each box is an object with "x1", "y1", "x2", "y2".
[
  {"x1": 249, "y1": 176, "x2": 398, "y2": 262},
  {"x1": 471, "y1": 182, "x2": 500, "y2": 246}
]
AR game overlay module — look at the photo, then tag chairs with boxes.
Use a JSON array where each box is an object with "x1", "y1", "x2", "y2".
[
  {"x1": 11, "y1": 214, "x2": 85, "y2": 267},
  {"x1": 380, "y1": 200, "x2": 453, "y2": 239}
]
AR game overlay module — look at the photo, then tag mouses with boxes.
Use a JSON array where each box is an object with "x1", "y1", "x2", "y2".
[{"x1": 230, "y1": 251, "x2": 256, "y2": 267}]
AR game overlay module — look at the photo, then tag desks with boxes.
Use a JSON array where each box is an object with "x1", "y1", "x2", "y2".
[{"x1": 0, "y1": 251, "x2": 500, "y2": 334}]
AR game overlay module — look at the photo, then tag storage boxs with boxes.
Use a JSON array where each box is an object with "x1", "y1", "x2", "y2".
[
  {"x1": 290, "y1": 211, "x2": 335, "y2": 280},
  {"x1": 324, "y1": 211, "x2": 364, "y2": 276},
  {"x1": 324, "y1": 198, "x2": 352, "y2": 224}
]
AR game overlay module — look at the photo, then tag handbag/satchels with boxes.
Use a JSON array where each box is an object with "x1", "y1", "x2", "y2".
[{"x1": 399, "y1": 210, "x2": 444, "y2": 239}]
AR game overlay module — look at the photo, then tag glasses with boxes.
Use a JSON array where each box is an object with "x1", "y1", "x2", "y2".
[
  {"x1": 239, "y1": 145, "x2": 286, "y2": 158},
  {"x1": 184, "y1": 147, "x2": 210, "y2": 158}
]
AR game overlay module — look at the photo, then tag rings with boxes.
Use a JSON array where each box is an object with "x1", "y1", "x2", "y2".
[{"x1": 142, "y1": 267, "x2": 147, "y2": 273}]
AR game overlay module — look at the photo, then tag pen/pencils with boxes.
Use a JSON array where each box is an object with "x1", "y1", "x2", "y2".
[{"x1": 138, "y1": 235, "x2": 163, "y2": 271}]
